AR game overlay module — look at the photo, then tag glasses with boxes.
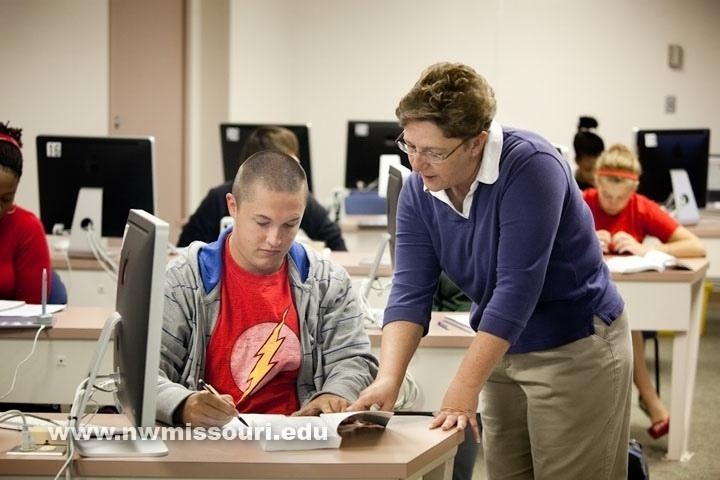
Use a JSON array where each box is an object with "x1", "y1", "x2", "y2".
[{"x1": 394, "y1": 128, "x2": 479, "y2": 165}]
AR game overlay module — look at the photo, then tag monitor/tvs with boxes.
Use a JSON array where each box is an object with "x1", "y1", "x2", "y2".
[
  {"x1": 362, "y1": 163, "x2": 413, "y2": 309},
  {"x1": 66, "y1": 210, "x2": 170, "y2": 456},
  {"x1": 635, "y1": 127, "x2": 710, "y2": 228},
  {"x1": 345, "y1": 121, "x2": 411, "y2": 189},
  {"x1": 34, "y1": 134, "x2": 156, "y2": 259},
  {"x1": 219, "y1": 122, "x2": 312, "y2": 194}
]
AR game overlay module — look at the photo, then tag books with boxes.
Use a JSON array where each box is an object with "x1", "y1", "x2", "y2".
[
  {"x1": 0, "y1": 299, "x2": 65, "y2": 323},
  {"x1": 605, "y1": 250, "x2": 694, "y2": 275},
  {"x1": 257, "y1": 410, "x2": 394, "y2": 449}
]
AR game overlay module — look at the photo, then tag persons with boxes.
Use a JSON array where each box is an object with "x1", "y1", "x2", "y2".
[
  {"x1": 430, "y1": 270, "x2": 483, "y2": 480},
  {"x1": 581, "y1": 141, "x2": 707, "y2": 441},
  {"x1": 175, "y1": 126, "x2": 353, "y2": 252},
  {"x1": 151, "y1": 149, "x2": 422, "y2": 433},
  {"x1": 0, "y1": 119, "x2": 68, "y2": 414},
  {"x1": 344, "y1": 59, "x2": 633, "y2": 480},
  {"x1": 574, "y1": 116, "x2": 606, "y2": 193}
]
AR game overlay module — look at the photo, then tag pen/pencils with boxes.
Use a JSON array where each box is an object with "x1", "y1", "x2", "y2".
[{"x1": 197, "y1": 378, "x2": 251, "y2": 428}]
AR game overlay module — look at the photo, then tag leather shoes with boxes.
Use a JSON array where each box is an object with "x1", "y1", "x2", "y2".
[{"x1": 646, "y1": 416, "x2": 671, "y2": 441}]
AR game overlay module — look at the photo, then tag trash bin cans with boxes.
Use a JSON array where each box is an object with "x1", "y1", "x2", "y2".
[{"x1": 657, "y1": 280, "x2": 711, "y2": 335}]
